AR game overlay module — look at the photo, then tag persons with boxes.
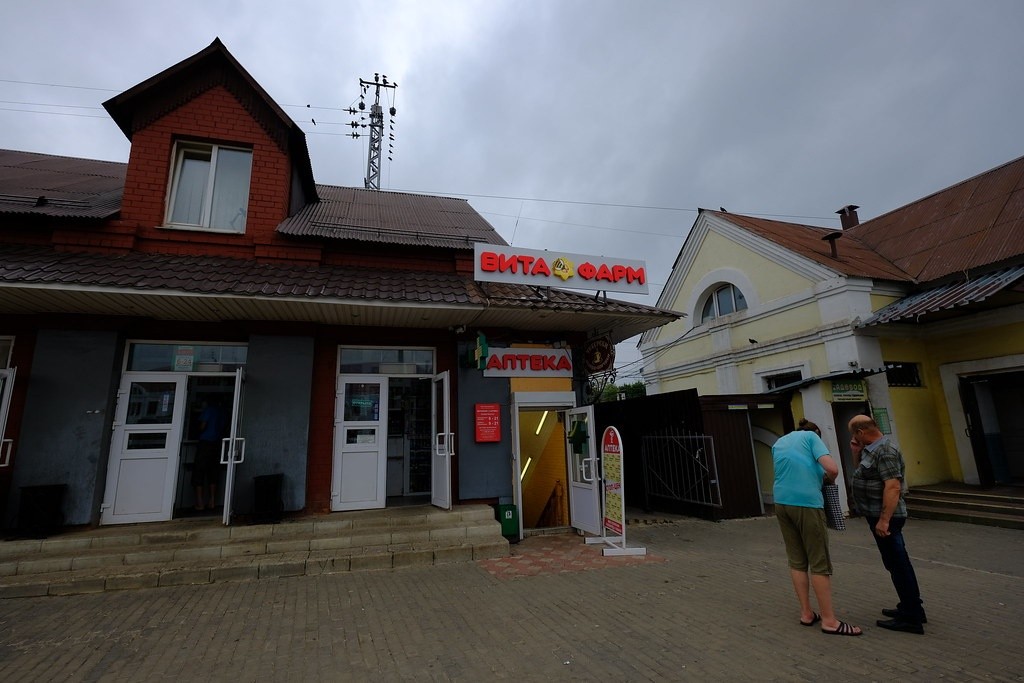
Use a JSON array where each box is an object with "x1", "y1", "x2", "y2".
[
  {"x1": 771, "y1": 417, "x2": 864, "y2": 637},
  {"x1": 848, "y1": 414, "x2": 929, "y2": 636},
  {"x1": 183, "y1": 392, "x2": 227, "y2": 513}
]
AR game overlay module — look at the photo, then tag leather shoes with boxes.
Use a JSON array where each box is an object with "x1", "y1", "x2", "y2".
[
  {"x1": 882, "y1": 607, "x2": 927, "y2": 623},
  {"x1": 877, "y1": 615, "x2": 924, "y2": 635}
]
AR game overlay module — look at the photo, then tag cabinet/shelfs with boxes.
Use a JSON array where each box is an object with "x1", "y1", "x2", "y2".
[
  {"x1": 403, "y1": 434, "x2": 431, "y2": 497},
  {"x1": 387, "y1": 434, "x2": 404, "y2": 496}
]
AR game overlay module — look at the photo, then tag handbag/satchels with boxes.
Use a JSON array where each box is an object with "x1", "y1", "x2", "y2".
[{"x1": 822, "y1": 473, "x2": 847, "y2": 531}]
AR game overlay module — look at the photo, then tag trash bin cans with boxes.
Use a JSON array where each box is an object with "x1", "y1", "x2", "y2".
[
  {"x1": 246, "y1": 472, "x2": 285, "y2": 525},
  {"x1": 14, "y1": 483, "x2": 71, "y2": 539},
  {"x1": 494, "y1": 503, "x2": 521, "y2": 545}
]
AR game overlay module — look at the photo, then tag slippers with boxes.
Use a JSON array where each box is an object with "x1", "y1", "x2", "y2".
[
  {"x1": 800, "y1": 611, "x2": 821, "y2": 626},
  {"x1": 821, "y1": 620, "x2": 862, "y2": 635}
]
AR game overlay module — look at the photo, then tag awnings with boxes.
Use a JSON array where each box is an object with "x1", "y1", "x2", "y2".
[{"x1": 856, "y1": 265, "x2": 1024, "y2": 331}]
